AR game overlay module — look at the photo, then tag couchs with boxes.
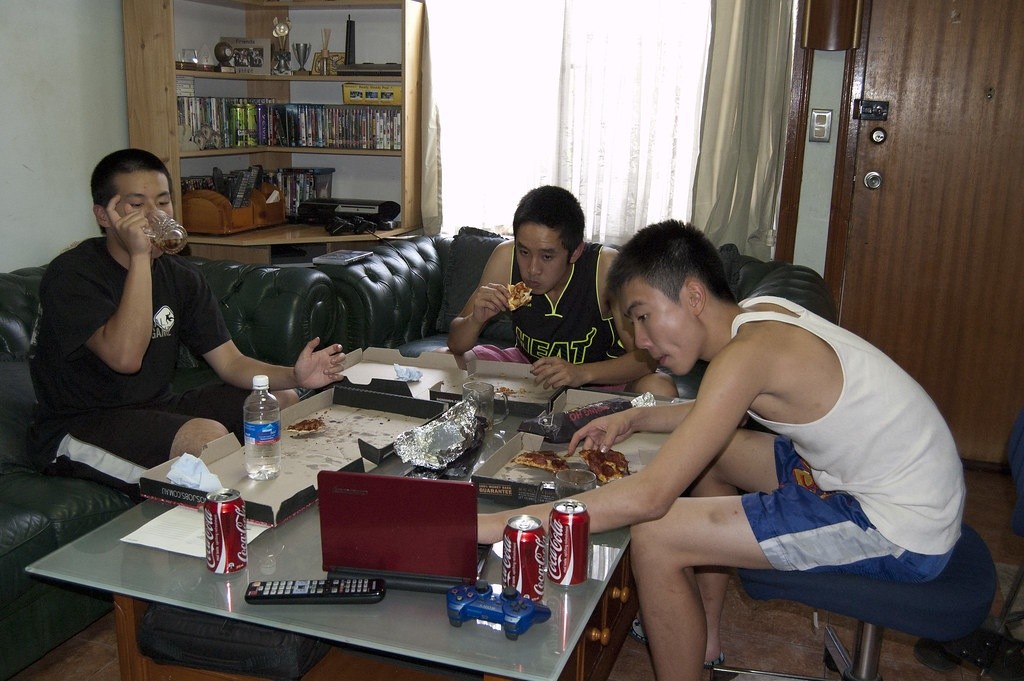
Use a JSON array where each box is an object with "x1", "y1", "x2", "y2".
[
  {"x1": 0, "y1": 256, "x2": 340, "y2": 681},
  {"x1": 311, "y1": 232, "x2": 835, "y2": 437}
]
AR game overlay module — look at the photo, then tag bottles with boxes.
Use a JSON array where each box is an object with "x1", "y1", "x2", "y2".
[
  {"x1": 320, "y1": 49, "x2": 330, "y2": 76},
  {"x1": 243, "y1": 375, "x2": 281, "y2": 481}
]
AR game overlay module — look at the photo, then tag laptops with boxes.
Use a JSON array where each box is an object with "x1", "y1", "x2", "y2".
[{"x1": 318, "y1": 470, "x2": 496, "y2": 592}]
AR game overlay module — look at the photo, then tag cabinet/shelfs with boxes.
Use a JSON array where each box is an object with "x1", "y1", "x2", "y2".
[{"x1": 122, "y1": 0, "x2": 425, "y2": 264}]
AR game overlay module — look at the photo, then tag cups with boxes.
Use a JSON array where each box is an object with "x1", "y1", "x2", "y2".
[
  {"x1": 554, "y1": 469, "x2": 596, "y2": 500},
  {"x1": 141, "y1": 210, "x2": 188, "y2": 255},
  {"x1": 462, "y1": 381, "x2": 509, "y2": 431}
]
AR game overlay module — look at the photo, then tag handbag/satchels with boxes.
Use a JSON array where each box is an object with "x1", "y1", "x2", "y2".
[{"x1": 136, "y1": 601, "x2": 334, "y2": 681}]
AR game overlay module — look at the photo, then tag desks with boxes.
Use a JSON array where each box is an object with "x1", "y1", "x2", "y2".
[{"x1": 24, "y1": 381, "x2": 674, "y2": 680}]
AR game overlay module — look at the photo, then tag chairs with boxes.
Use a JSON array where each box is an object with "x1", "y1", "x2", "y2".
[{"x1": 710, "y1": 406, "x2": 1024, "y2": 681}]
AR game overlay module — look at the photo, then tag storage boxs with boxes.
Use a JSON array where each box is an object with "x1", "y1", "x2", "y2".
[
  {"x1": 341, "y1": 82, "x2": 402, "y2": 106},
  {"x1": 139, "y1": 346, "x2": 696, "y2": 527}
]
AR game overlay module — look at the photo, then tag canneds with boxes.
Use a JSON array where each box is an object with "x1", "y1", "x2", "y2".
[
  {"x1": 204, "y1": 488, "x2": 248, "y2": 575},
  {"x1": 502, "y1": 515, "x2": 547, "y2": 602},
  {"x1": 547, "y1": 499, "x2": 591, "y2": 585},
  {"x1": 229, "y1": 102, "x2": 258, "y2": 148}
]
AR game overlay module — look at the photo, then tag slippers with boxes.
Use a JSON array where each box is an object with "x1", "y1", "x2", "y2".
[{"x1": 632, "y1": 619, "x2": 724, "y2": 667}]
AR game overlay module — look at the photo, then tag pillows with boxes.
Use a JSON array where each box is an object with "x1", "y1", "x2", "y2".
[{"x1": 436, "y1": 227, "x2": 515, "y2": 343}]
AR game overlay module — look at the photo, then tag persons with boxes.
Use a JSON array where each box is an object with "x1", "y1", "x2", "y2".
[
  {"x1": 34, "y1": 148, "x2": 346, "y2": 469},
  {"x1": 234, "y1": 48, "x2": 264, "y2": 67},
  {"x1": 478, "y1": 220, "x2": 965, "y2": 681},
  {"x1": 430, "y1": 185, "x2": 679, "y2": 398}
]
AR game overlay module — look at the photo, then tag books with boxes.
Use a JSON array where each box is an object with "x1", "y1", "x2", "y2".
[
  {"x1": 181, "y1": 176, "x2": 216, "y2": 193},
  {"x1": 178, "y1": 95, "x2": 276, "y2": 148},
  {"x1": 313, "y1": 250, "x2": 373, "y2": 265},
  {"x1": 276, "y1": 103, "x2": 402, "y2": 150},
  {"x1": 264, "y1": 168, "x2": 336, "y2": 216},
  {"x1": 212, "y1": 165, "x2": 262, "y2": 207}
]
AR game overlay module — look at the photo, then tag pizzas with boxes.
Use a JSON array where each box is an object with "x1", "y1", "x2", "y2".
[
  {"x1": 506, "y1": 281, "x2": 532, "y2": 311},
  {"x1": 511, "y1": 450, "x2": 571, "y2": 472},
  {"x1": 287, "y1": 417, "x2": 325, "y2": 433},
  {"x1": 580, "y1": 448, "x2": 632, "y2": 483}
]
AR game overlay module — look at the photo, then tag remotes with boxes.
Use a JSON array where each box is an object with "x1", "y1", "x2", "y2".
[{"x1": 245, "y1": 577, "x2": 387, "y2": 605}]
